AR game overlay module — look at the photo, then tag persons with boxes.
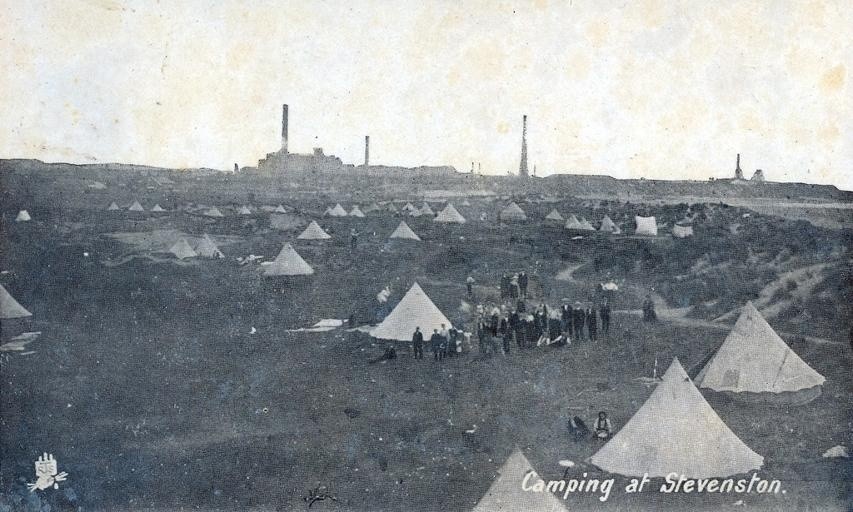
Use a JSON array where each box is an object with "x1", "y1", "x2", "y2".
[
  {"x1": 566, "y1": 411, "x2": 590, "y2": 443},
  {"x1": 430, "y1": 297, "x2": 611, "y2": 360},
  {"x1": 516, "y1": 270, "x2": 528, "y2": 298},
  {"x1": 499, "y1": 270, "x2": 510, "y2": 299},
  {"x1": 369, "y1": 345, "x2": 397, "y2": 365},
  {"x1": 349, "y1": 227, "x2": 358, "y2": 250},
  {"x1": 410, "y1": 327, "x2": 422, "y2": 359},
  {"x1": 590, "y1": 409, "x2": 612, "y2": 440},
  {"x1": 465, "y1": 278, "x2": 475, "y2": 293},
  {"x1": 508, "y1": 269, "x2": 519, "y2": 296},
  {"x1": 641, "y1": 295, "x2": 657, "y2": 321}
]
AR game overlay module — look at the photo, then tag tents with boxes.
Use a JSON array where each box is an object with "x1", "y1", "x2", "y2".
[
  {"x1": 585, "y1": 354, "x2": 764, "y2": 479},
  {"x1": 197, "y1": 233, "x2": 226, "y2": 260},
  {"x1": 167, "y1": 235, "x2": 196, "y2": 261},
  {"x1": 0, "y1": 280, "x2": 31, "y2": 322},
  {"x1": 262, "y1": 242, "x2": 315, "y2": 276},
  {"x1": 469, "y1": 444, "x2": 570, "y2": 511},
  {"x1": 695, "y1": 302, "x2": 826, "y2": 398},
  {"x1": 365, "y1": 282, "x2": 455, "y2": 339},
  {"x1": 14, "y1": 209, "x2": 31, "y2": 221},
  {"x1": 107, "y1": 200, "x2": 695, "y2": 243}
]
